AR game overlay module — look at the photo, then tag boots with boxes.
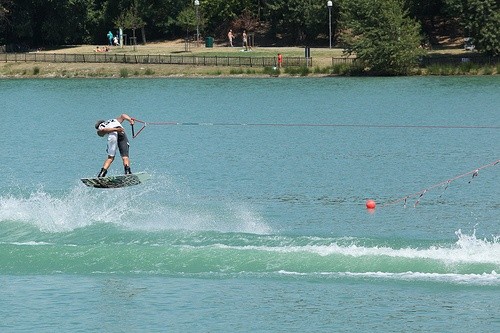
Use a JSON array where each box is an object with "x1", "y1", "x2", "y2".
[
  {"x1": 124, "y1": 164, "x2": 132, "y2": 174},
  {"x1": 98, "y1": 168, "x2": 107, "y2": 177}
]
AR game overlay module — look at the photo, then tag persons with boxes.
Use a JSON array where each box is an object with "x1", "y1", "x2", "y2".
[
  {"x1": 113, "y1": 35, "x2": 120, "y2": 46},
  {"x1": 95, "y1": 113, "x2": 134, "y2": 178},
  {"x1": 93, "y1": 46, "x2": 113, "y2": 52},
  {"x1": 228, "y1": 29, "x2": 235, "y2": 47},
  {"x1": 242, "y1": 30, "x2": 247, "y2": 47},
  {"x1": 107, "y1": 31, "x2": 114, "y2": 46}
]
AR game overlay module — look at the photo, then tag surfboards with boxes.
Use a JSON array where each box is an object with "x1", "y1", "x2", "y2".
[{"x1": 79, "y1": 171, "x2": 152, "y2": 189}]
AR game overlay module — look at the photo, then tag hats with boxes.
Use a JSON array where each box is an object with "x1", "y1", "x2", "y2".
[{"x1": 94, "y1": 120, "x2": 106, "y2": 130}]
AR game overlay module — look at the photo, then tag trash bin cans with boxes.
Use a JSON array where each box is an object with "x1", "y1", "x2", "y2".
[{"x1": 205, "y1": 37, "x2": 213, "y2": 48}]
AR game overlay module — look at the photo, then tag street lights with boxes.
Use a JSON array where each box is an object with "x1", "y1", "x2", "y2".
[
  {"x1": 327, "y1": 1, "x2": 332, "y2": 51},
  {"x1": 194, "y1": 0, "x2": 199, "y2": 48}
]
